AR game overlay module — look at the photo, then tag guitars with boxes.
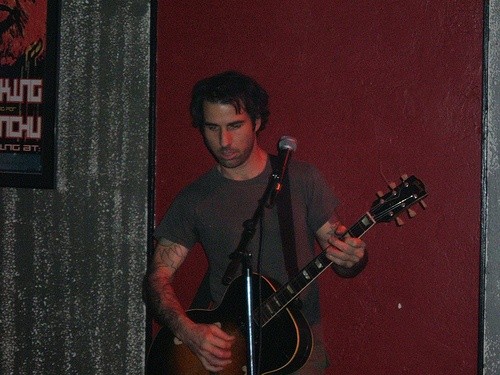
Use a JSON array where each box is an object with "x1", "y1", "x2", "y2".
[{"x1": 144, "y1": 172, "x2": 429, "y2": 375}]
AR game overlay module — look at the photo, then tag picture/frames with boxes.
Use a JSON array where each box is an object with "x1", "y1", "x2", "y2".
[{"x1": 0, "y1": 0, "x2": 62, "y2": 189}]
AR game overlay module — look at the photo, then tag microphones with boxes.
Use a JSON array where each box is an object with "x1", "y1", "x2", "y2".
[{"x1": 263, "y1": 136, "x2": 298, "y2": 210}]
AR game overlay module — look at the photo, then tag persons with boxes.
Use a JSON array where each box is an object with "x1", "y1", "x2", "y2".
[{"x1": 143, "y1": 71, "x2": 369, "y2": 374}]
